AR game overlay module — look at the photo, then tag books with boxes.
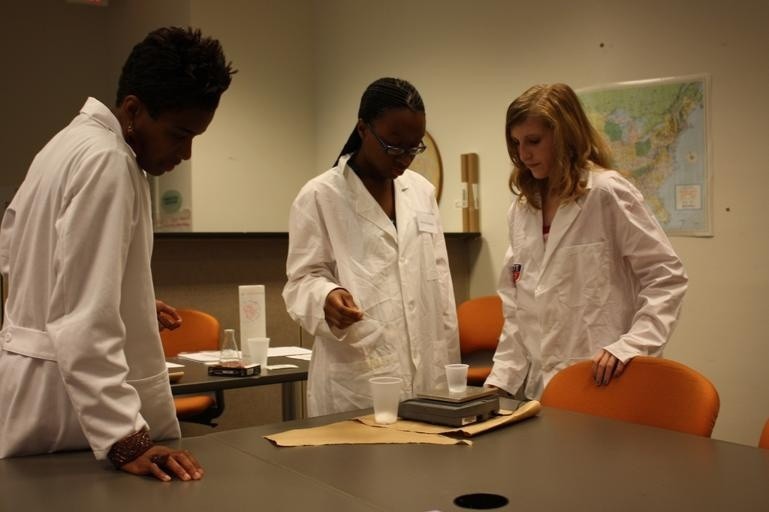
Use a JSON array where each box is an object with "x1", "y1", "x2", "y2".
[{"x1": 166, "y1": 360, "x2": 186, "y2": 381}]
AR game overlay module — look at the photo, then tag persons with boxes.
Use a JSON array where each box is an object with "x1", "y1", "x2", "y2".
[
  {"x1": 0, "y1": 26, "x2": 242, "y2": 484},
  {"x1": 279, "y1": 76, "x2": 461, "y2": 420},
  {"x1": 483, "y1": 81, "x2": 688, "y2": 404}
]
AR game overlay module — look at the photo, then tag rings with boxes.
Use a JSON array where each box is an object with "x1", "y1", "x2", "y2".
[{"x1": 160, "y1": 451, "x2": 171, "y2": 464}]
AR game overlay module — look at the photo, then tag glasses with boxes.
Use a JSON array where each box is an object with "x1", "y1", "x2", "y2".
[{"x1": 364, "y1": 119, "x2": 427, "y2": 157}]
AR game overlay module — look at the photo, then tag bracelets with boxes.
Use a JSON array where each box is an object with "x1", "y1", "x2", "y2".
[{"x1": 108, "y1": 426, "x2": 156, "y2": 470}]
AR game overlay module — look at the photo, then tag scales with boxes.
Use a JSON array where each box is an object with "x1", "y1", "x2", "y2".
[{"x1": 397, "y1": 385, "x2": 500, "y2": 426}]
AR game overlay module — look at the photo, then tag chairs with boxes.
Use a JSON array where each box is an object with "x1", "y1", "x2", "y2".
[
  {"x1": 539, "y1": 355, "x2": 719, "y2": 438},
  {"x1": 456, "y1": 296, "x2": 503, "y2": 383},
  {"x1": 159, "y1": 309, "x2": 224, "y2": 427}
]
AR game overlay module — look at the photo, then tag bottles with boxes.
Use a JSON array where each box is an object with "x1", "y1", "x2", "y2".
[{"x1": 219, "y1": 328, "x2": 240, "y2": 364}]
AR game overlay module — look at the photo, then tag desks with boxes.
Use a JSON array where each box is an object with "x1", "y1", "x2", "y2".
[
  {"x1": 207, "y1": 398, "x2": 769, "y2": 512},
  {"x1": 0, "y1": 436, "x2": 389, "y2": 512},
  {"x1": 165, "y1": 345, "x2": 309, "y2": 421}
]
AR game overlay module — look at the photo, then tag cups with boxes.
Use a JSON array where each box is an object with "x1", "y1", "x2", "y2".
[
  {"x1": 367, "y1": 376, "x2": 404, "y2": 425},
  {"x1": 444, "y1": 363, "x2": 470, "y2": 393},
  {"x1": 247, "y1": 337, "x2": 271, "y2": 370}
]
